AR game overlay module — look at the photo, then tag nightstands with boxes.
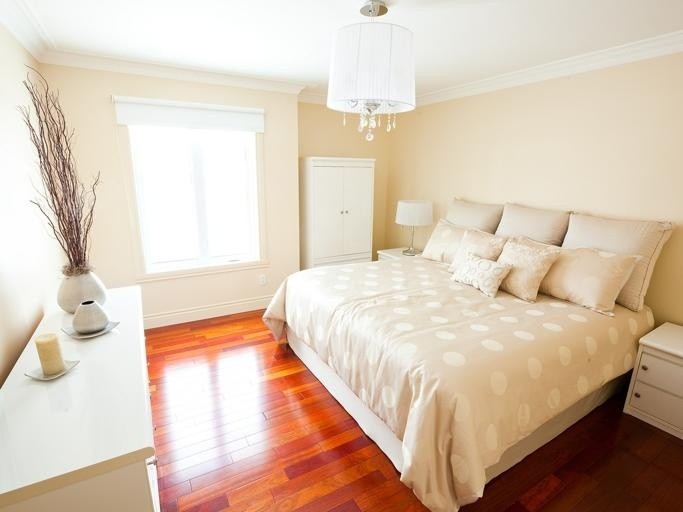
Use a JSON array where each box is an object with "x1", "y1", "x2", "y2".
[
  {"x1": 622, "y1": 321, "x2": 683, "y2": 440},
  {"x1": 377, "y1": 246, "x2": 418, "y2": 261}
]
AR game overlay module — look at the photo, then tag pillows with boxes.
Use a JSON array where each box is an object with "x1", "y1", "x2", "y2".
[
  {"x1": 493, "y1": 202, "x2": 571, "y2": 247},
  {"x1": 426, "y1": 199, "x2": 506, "y2": 235},
  {"x1": 444, "y1": 228, "x2": 507, "y2": 275},
  {"x1": 421, "y1": 218, "x2": 466, "y2": 268},
  {"x1": 451, "y1": 254, "x2": 514, "y2": 298},
  {"x1": 562, "y1": 210, "x2": 674, "y2": 313},
  {"x1": 496, "y1": 237, "x2": 564, "y2": 303},
  {"x1": 539, "y1": 247, "x2": 641, "y2": 317}
]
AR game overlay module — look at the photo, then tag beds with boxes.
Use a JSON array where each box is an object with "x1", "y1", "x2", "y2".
[{"x1": 262, "y1": 251, "x2": 682, "y2": 512}]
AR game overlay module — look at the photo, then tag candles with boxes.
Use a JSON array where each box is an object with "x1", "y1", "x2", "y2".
[{"x1": 33, "y1": 333, "x2": 66, "y2": 376}]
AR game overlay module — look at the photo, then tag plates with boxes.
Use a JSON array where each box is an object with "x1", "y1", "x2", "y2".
[
  {"x1": 24, "y1": 359, "x2": 80, "y2": 380},
  {"x1": 59, "y1": 317, "x2": 119, "y2": 340}
]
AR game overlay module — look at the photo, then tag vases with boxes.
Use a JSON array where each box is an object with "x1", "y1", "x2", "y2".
[
  {"x1": 70, "y1": 297, "x2": 106, "y2": 333},
  {"x1": 57, "y1": 271, "x2": 111, "y2": 313}
]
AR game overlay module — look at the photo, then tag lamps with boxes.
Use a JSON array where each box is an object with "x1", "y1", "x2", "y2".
[
  {"x1": 396, "y1": 199, "x2": 436, "y2": 254},
  {"x1": 325, "y1": 1, "x2": 418, "y2": 141}
]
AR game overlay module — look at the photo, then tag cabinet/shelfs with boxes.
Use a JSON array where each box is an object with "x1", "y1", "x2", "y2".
[
  {"x1": 0, "y1": 288, "x2": 163, "y2": 512},
  {"x1": 301, "y1": 156, "x2": 376, "y2": 270}
]
